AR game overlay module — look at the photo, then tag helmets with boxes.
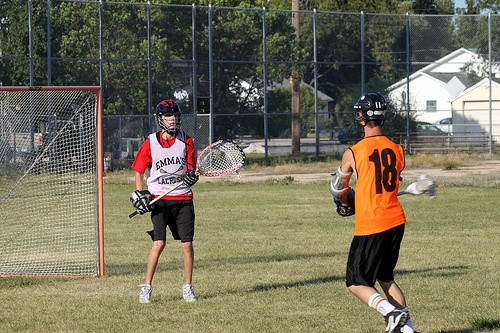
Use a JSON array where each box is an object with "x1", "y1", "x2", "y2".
[
  {"x1": 354, "y1": 93, "x2": 387, "y2": 125},
  {"x1": 155, "y1": 99, "x2": 182, "y2": 135}
]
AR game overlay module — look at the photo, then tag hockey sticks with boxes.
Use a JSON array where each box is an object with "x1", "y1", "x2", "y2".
[
  {"x1": 341, "y1": 177, "x2": 437, "y2": 213},
  {"x1": 128, "y1": 140, "x2": 247, "y2": 219}
]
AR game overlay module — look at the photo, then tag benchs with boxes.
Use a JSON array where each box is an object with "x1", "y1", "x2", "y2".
[{"x1": 395, "y1": 131, "x2": 497, "y2": 155}]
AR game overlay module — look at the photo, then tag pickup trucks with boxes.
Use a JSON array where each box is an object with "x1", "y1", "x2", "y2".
[{"x1": 9, "y1": 118, "x2": 82, "y2": 163}]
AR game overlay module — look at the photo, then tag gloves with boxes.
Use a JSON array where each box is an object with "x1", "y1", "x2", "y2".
[
  {"x1": 184, "y1": 170, "x2": 199, "y2": 186},
  {"x1": 333, "y1": 190, "x2": 355, "y2": 217},
  {"x1": 130, "y1": 191, "x2": 154, "y2": 214}
]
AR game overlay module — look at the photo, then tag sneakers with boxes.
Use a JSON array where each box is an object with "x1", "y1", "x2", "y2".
[
  {"x1": 182, "y1": 285, "x2": 197, "y2": 303},
  {"x1": 139, "y1": 284, "x2": 152, "y2": 304},
  {"x1": 383, "y1": 308, "x2": 405, "y2": 333}
]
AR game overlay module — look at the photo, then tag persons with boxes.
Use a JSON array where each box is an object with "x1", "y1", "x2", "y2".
[
  {"x1": 330, "y1": 92, "x2": 421, "y2": 333},
  {"x1": 131, "y1": 99, "x2": 198, "y2": 304}
]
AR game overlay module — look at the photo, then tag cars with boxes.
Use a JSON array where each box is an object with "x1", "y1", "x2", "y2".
[{"x1": 338, "y1": 117, "x2": 453, "y2": 144}]
